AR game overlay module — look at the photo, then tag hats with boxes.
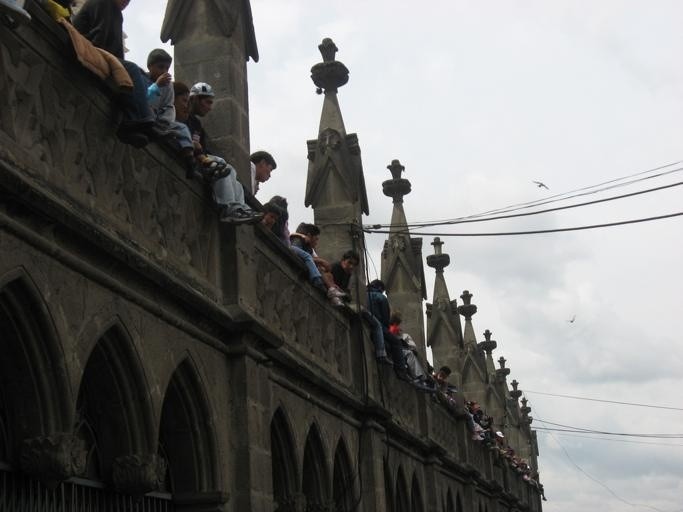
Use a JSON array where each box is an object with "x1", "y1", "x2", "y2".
[
  {"x1": 145, "y1": 48, "x2": 173, "y2": 67},
  {"x1": 187, "y1": 81, "x2": 216, "y2": 99},
  {"x1": 443, "y1": 383, "x2": 459, "y2": 395},
  {"x1": 438, "y1": 365, "x2": 451, "y2": 377}
]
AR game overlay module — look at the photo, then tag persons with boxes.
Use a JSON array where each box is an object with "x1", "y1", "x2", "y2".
[
  {"x1": 76, "y1": 1, "x2": 264, "y2": 225},
  {"x1": 250, "y1": 152, "x2": 547, "y2": 502}
]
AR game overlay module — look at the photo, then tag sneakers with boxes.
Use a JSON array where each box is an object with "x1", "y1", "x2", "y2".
[
  {"x1": 325, "y1": 286, "x2": 348, "y2": 310},
  {"x1": 219, "y1": 205, "x2": 265, "y2": 226},
  {"x1": 184, "y1": 160, "x2": 231, "y2": 184},
  {"x1": 471, "y1": 424, "x2": 531, "y2": 484},
  {"x1": 114, "y1": 115, "x2": 156, "y2": 149},
  {"x1": 376, "y1": 352, "x2": 394, "y2": 370}
]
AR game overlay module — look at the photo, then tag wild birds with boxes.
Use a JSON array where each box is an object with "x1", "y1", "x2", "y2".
[
  {"x1": 532, "y1": 180, "x2": 549, "y2": 191},
  {"x1": 566, "y1": 314, "x2": 576, "y2": 324}
]
